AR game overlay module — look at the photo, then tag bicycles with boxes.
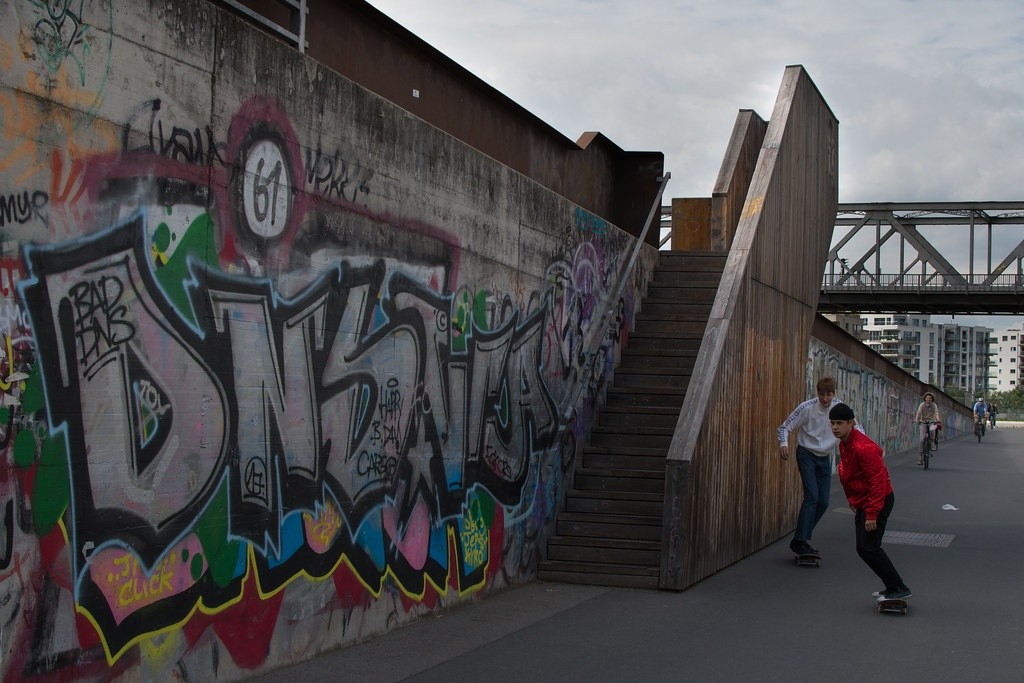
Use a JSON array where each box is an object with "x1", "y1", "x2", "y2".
[
  {"x1": 987, "y1": 411, "x2": 999, "y2": 430},
  {"x1": 914, "y1": 420, "x2": 941, "y2": 471},
  {"x1": 972, "y1": 414, "x2": 989, "y2": 443}
]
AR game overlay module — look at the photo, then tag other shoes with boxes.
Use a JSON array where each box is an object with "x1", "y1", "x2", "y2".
[
  {"x1": 919, "y1": 454, "x2": 923, "y2": 464},
  {"x1": 790, "y1": 539, "x2": 820, "y2": 555},
  {"x1": 931, "y1": 441, "x2": 936, "y2": 451}
]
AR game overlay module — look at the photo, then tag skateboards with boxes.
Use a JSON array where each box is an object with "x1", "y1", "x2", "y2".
[
  {"x1": 877, "y1": 598, "x2": 909, "y2": 615},
  {"x1": 794, "y1": 554, "x2": 823, "y2": 567}
]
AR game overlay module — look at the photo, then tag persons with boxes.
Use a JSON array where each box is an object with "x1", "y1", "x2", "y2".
[
  {"x1": 974, "y1": 398, "x2": 987, "y2": 436},
  {"x1": 989, "y1": 405, "x2": 998, "y2": 426},
  {"x1": 829, "y1": 403, "x2": 912, "y2": 601},
  {"x1": 916, "y1": 393, "x2": 941, "y2": 465},
  {"x1": 778, "y1": 376, "x2": 865, "y2": 555}
]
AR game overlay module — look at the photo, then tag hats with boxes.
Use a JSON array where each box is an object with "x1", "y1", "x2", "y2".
[
  {"x1": 979, "y1": 398, "x2": 984, "y2": 402},
  {"x1": 829, "y1": 403, "x2": 854, "y2": 420}
]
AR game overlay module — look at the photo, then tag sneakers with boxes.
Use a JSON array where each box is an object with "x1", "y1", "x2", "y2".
[
  {"x1": 871, "y1": 588, "x2": 889, "y2": 597},
  {"x1": 877, "y1": 589, "x2": 913, "y2": 602}
]
